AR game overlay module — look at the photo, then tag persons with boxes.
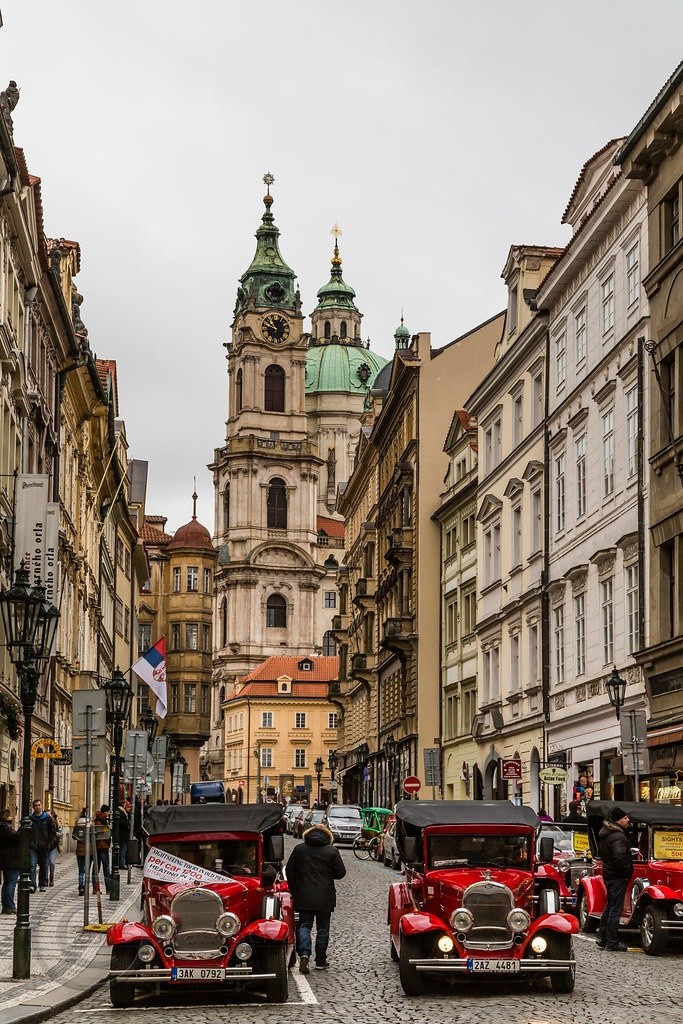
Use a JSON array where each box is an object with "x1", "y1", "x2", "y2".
[
  {"x1": 220, "y1": 841, "x2": 278, "y2": 884},
  {"x1": 0, "y1": 798, "x2": 181, "y2": 918},
  {"x1": 286, "y1": 825, "x2": 348, "y2": 974},
  {"x1": 266, "y1": 797, "x2": 361, "y2": 810},
  {"x1": 475, "y1": 801, "x2": 632, "y2": 951}
]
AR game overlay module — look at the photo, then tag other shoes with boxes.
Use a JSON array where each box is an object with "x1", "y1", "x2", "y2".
[
  {"x1": 40, "y1": 886, "x2": 46, "y2": 892},
  {"x1": 7, "y1": 908, "x2": 17, "y2": 913},
  {"x1": 94, "y1": 889, "x2": 102, "y2": 894},
  {"x1": 299, "y1": 953, "x2": 310, "y2": 974},
  {"x1": 595, "y1": 938, "x2": 605, "y2": 948},
  {"x1": 121, "y1": 865, "x2": 128, "y2": 869},
  {"x1": 315, "y1": 960, "x2": 329, "y2": 969},
  {"x1": 79, "y1": 889, "x2": 84, "y2": 896},
  {"x1": 30, "y1": 886, "x2": 35, "y2": 893},
  {"x1": 2, "y1": 908, "x2": 7, "y2": 913},
  {"x1": 606, "y1": 944, "x2": 627, "y2": 952}
]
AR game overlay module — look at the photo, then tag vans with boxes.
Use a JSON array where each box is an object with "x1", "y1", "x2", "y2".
[{"x1": 190, "y1": 782, "x2": 225, "y2": 805}]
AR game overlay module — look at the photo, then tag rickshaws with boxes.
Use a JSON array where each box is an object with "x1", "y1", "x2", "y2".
[{"x1": 352, "y1": 808, "x2": 393, "y2": 861}]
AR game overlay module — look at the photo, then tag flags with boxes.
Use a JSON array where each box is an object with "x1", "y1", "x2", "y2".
[{"x1": 131, "y1": 634, "x2": 168, "y2": 719}]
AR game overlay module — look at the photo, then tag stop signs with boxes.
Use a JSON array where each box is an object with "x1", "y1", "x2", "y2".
[{"x1": 403, "y1": 776, "x2": 421, "y2": 794}]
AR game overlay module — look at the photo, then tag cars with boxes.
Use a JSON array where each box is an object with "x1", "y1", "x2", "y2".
[
  {"x1": 576, "y1": 800, "x2": 683, "y2": 957},
  {"x1": 107, "y1": 803, "x2": 296, "y2": 1009},
  {"x1": 386, "y1": 799, "x2": 580, "y2": 996},
  {"x1": 383, "y1": 820, "x2": 399, "y2": 870},
  {"x1": 293, "y1": 809, "x2": 312, "y2": 839},
  {"x1": 286, "y1": 809, "x2": 301, "y2": 835},
  {"x1": 302, "y1": 811, "x2": 326, "y2": 833},
  {"x1": 520, "y1": 820, "x2": 593, "y2": 911}
]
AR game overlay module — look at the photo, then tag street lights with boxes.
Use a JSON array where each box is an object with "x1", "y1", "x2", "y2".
[
  {"x1": 327, "y1": 753, "x2": 339, "y2": 804},
  {"x1": 138, "y1": 705, "x2": 159, "y2": 804},
  {"x1": 161, "y1": 726, "x2": 172, "y2": 801},
  {"x1": 101, "y1": 664, "x2": 136, "y2": 900},
  {"x1": 166, "y1": 741, "x2": 189, "y2": 805},
  {"x1": 313, "y1": 757, "x2": 325, "y2": 810},
  {"x1": 0, "y1": 557, "x2": 62, "y2": 979}
]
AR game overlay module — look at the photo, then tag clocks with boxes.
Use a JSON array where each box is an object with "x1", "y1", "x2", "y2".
[{"x1": 260, "y1": 314, "x2": 291, "y2": 345}]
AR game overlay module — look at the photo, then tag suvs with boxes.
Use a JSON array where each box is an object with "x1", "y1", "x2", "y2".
[
  {"x1": 284, "y1": 805, "x2": 304, "y2": 818},
  {"x1": 321, "y1": 802, "x2": 365, "y2": 845}
]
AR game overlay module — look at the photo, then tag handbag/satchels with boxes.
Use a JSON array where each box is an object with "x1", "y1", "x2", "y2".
[{"x1": 50, "y1": 882, "x2": 54, "y2": 886}]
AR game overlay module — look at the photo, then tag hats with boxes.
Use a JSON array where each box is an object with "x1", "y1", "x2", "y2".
[
  {"x1": 125, "y1": 800, "x2": 132, "y2": 808},
  {"x1": 100, "y1": 805, "x2": 110, "y2": 813},
  {"x1": 611, "y1": 808, "x2": 627, "y2": 822}
]
